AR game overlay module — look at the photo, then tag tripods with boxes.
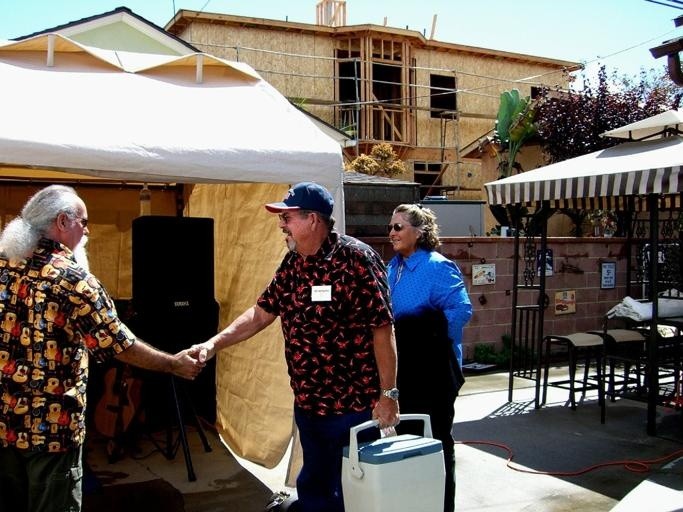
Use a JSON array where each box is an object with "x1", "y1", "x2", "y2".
[{"x1": 129, "y1": 314, "x2": 211, "y2": 485}]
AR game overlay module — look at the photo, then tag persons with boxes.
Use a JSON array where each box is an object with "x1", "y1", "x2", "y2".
[
  {"x1": 378, "y1": 203, "x2": 474, "y2": 511},
  {"x1": 183, "y1": 181, "x2": 401, "y2": 512},
  {"x1": 0, "y1": 184, "x2": 209, "y2": 512}
]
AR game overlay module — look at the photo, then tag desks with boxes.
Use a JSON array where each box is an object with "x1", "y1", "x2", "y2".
[{"x1": 598, "y1": 313, "x2": 682, "y2": 437}]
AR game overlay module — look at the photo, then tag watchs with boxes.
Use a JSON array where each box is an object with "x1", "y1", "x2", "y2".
[{"x1": 379, "y1": 386, "x2": 399, "y2": 401}]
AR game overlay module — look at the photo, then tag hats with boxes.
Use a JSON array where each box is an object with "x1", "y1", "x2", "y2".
[{"x1": 265, "y1": 181, "x2": 334, "y2": 215}]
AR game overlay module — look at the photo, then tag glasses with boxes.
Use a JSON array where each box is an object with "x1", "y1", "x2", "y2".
[
  {"x1": 388, "y1": 223, "x2": 404, "y2": 232},
  {"x1": 279, "y1": 214, "x2": 289, "y2": 223},
  {"x1": 76, "y1": 214, "x2": 88, "y2": 226}
]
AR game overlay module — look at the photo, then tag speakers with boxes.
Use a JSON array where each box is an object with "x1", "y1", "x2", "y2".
[{"x1": 130, "y1": 215, "x2": 216, "y2": 309}]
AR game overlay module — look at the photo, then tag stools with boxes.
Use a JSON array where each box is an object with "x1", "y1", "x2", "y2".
[{"x1": 540, "y1": 324, "x2": 683, "y2": 423}]
